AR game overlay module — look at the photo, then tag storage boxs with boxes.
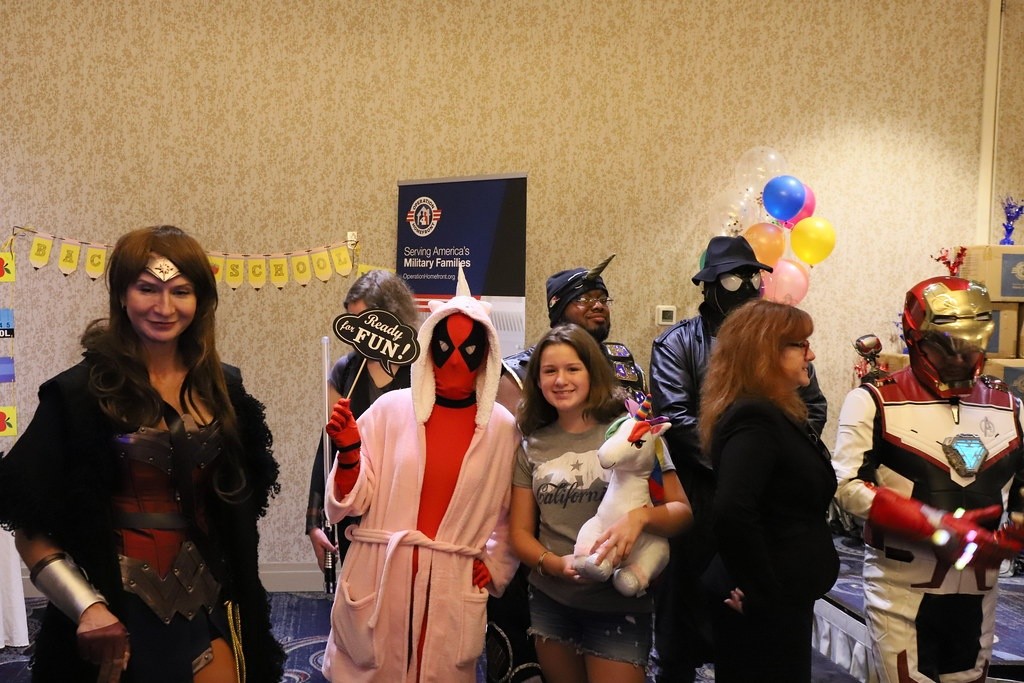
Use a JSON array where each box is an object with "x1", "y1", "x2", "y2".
[{"x1": 875, "y1": 245, "x2": 1024, "y2": 400}]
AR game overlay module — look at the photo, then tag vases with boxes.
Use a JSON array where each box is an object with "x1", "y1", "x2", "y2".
[{"x1": 999, "y1": 223, "x2": 1014, "y2": 245}]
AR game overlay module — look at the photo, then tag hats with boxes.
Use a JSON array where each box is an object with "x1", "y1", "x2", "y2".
[
  {"x1": 691, "y1": 235, "x2": 773, "y2": 286},
  {"x1": 546, "y1": 253, "x2": 616, "y2": 327}
]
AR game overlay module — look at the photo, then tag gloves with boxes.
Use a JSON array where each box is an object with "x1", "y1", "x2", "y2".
[
  {"x1": 939, "y1": 505, "x2": 1024, "y2": 570},
  {"x1": 472, "y1": 558, "x2": 492, "y2": 589},
  {"x1": 326, "y1": 397, "x2": 361, "y2": 455}
]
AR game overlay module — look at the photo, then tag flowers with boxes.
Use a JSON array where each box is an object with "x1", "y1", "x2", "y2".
[
  {"x1": 930, "y1": 248, "x2": 967, "y2": 276},
  {"x1": 894, "y1": 313, "x2": 905, "y2": 343},
  {"x1": 997, "y1": 196, "x2": 1024, "y2": 225}
]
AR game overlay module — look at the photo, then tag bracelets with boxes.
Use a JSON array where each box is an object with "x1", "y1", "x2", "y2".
[{"x1": 536, "y1": 551, "x2": 554, "y2": 578}]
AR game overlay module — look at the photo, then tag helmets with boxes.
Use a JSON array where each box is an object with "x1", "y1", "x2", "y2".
[{"x1": 902, "y1": 276, "x2": 995, "y2": 399}]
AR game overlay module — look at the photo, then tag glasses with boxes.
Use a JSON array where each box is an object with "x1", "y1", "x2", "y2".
[
  {"x1": 572, "y1": 296, "x2": 615, "y2": 306},
  {"x1": 787, "y1": 340, "x2": 809, "y2": 357}
]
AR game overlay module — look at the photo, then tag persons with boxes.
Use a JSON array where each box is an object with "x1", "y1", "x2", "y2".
[
  {"x1": 830, "y1": 276, "x2": 1024, "y2": 683},
  {"x1": 650, "y1": 235, "x2": 840, "y2": 683},
  {"x1": 305, "y1": 254, "x2": 692, "y2": 683},
  {"x1": 0, "y1": 225, "x2": 287, "y2": 683}
]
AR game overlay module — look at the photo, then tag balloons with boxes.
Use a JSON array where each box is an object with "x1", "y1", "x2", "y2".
[{"x1": 700, "y1": 146, "x2": 836, "y2": 306}]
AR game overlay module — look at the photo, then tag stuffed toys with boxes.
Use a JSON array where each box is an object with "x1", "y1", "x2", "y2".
[{"x1": 572, "y1": 393, "x2": 671, "y2": 598}]
